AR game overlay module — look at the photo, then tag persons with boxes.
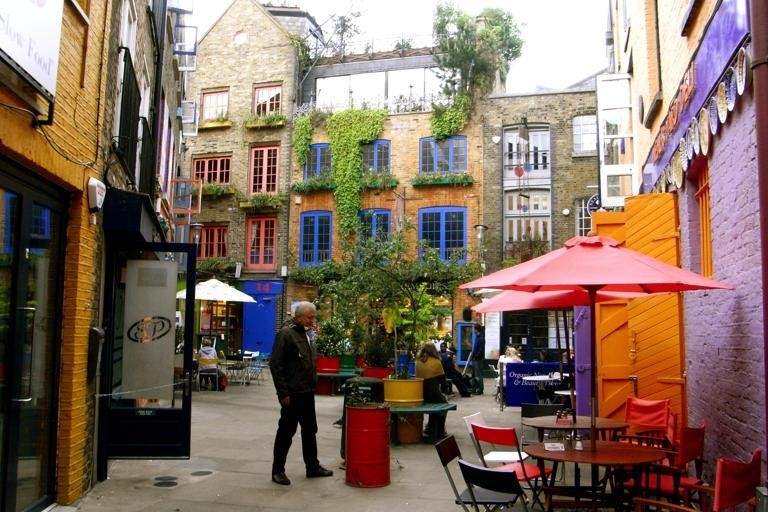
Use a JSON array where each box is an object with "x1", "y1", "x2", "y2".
[
  {"x1": 268, "y1": 302, "x2": 333, "y2": 485},
  {"x1": 415, "y1": 343, "x2": 448, "y2": 438},
  {"x1": 197, "y1": 338, "x2": 218, "y2": 391},
  {"x1": 492, "y1": 345, "x2": 575, "y2": 404},
  {"x1": 439, "y1": 343, "x2": 472, "y2": 398},
  {"x1": 471, "y1": 323, "x2": 485, "y2": 394}
]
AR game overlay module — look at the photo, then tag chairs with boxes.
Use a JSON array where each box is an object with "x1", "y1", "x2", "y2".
[
  {"x1": 434, "y1": 396, "x2": 763, "y2": 512},
  {"x1": 197, "y1": 350, "x2": 270, "y2": 392}
]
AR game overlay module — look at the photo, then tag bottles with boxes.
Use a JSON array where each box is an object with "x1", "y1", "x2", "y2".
[
  {"x1": 231, "y1": 351, "x2": 233, "y2": 356},
  {"x1": 575, "y1": 435, "x2": 585, "y2": 451},
  {"x1": 565, "y1": 435, "x2": 573, "y2": 450},
  {"x1": 549, "y1": 371, "x2": 553, "y2": 378},
  {"x1": 556, "y1": 409, "x2": 567, "y2": 424}
]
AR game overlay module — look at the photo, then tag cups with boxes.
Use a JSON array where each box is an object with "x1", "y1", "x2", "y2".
[{"x1": 238, "y1": 349, "x2": 241, "y2": 354}]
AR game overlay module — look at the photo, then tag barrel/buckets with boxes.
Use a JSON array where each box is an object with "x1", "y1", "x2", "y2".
[
  {"x1": 383, "y1": 376, "x2": 424, "y2": 404},
  {"x1": 344, "y1": 402, "x2": 391, "y2": 488},
  {"x1": 315, "y1": 353, "x2": 414, "y2": 392}
]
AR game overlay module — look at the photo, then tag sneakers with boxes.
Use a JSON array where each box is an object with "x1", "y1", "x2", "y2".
[
  {"x1": 272, "y1": 471, "x2": 291, "y2": 486},
  {"x1": 306, "y1": 463, "x2": 334, "y2": 479}
]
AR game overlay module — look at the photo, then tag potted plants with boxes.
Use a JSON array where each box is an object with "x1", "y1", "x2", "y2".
[{"x1": 283, "y1": 210, "x2": 487, "y2": 488}]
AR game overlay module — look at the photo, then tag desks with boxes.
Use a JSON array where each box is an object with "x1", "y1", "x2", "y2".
[{"x1": 522, "y1": 375, "x2": 563, "y2": 405}]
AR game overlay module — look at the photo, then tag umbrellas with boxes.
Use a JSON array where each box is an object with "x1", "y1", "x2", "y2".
[
  {"x1": 470, "y1": 288, "x2": 672, "y2": 423},
  {"x1": 176, "y1": 278, "x2": 258, "y2": 337},
  {"x1": 459, "y1": 233, "x2": 735, "y2": 454}
]
